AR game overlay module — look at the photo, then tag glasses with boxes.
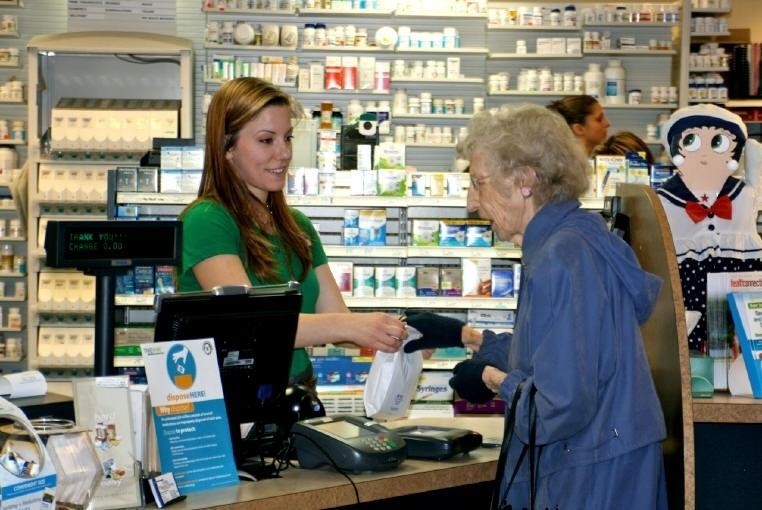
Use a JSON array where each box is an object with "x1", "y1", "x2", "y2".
[{"x1": 471, "y1": 160, "x2": 528, "y2": 192}]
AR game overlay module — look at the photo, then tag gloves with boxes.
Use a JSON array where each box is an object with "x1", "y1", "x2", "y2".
[
  {"x1": 401, "y1": 311, "x2": 465, "y2": 352},
  {"x1": 449, "y1": 360, "x2": 497, "y2": 405}
]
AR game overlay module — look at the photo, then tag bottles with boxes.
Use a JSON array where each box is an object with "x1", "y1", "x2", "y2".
[
  {"x1": 473, "y1": 97, "x2": 485, "y2": 113},
  {"x1": 487, "y1": 58, "x2": 626, "y2": 104},
  {"x1": 515, "y1": 39, "x2": 527, "y2": 56},
  {"x1": 582, "y1": 31, "x2": 600, "y2": 52},
  {"x1": 0, "y1": 13, "x2": 29, "y2": 365},
  {"x1": 206, "y1": 55, "x2": 300, "y2": 86},
  {"x1": 0, "y1": 418, "x2": 105, "y2": 510},
  {"x1": 395, "y1": 88, "x2": 465, "y2": 113},
  {"x1": 689, "y1": 0, "x2": 731, "y2": 102},
  {"x1": 648, "y1": 84, "x2": 678, "y2": 103},
  {"x1": 305, "y1": 100, "x2": 343, "y2": 132},
  {"x1": 347, "y1": 100, "x2": 391, "y2": 124},
  {"x1": 649, "y1": 39, "x2": 672, "y2": 51},
  {"x1": 644, "y1": 112, "x2": 670, "y2": 139},
  {"x1": 206, "y1": 18, "x2": 461, "y2": 50},
  {"x1": 393, "y1": 58, "x2": 444, "y2": 83},
  {"x1": 484, "y1": 3, "x2": 680, "y2": 30},
  {"x1": 628, "y1": 88, "x2": 642, "y2": 106},
  {"x1": 395, "y1": 126, "x2": 469, "y2": 144}
]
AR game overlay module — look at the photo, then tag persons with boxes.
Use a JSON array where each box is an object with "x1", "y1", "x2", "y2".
[
  {"x1": 173, "y1": 76, "x2": 407, "y2": 423},
  {"x1": 547, "y1": 93, "x2": 609, "y2": 159},
  {"x1": 400, "y1": 102, "x2": 670, "y2": 510},
  {"x1": 595, "y1": 128, "x2": 656, "y2": 178},
  {"x1": 655, "y1": 102, "x2": 762, "y2": 354}
]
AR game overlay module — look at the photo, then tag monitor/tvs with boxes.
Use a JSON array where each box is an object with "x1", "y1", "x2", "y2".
[{"x1": 154, "y1": 282, "x2": 302, "y2": 479}]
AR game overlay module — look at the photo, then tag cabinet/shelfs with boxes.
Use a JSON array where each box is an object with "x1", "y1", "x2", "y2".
[
  {"x1": 199, "y1": 5, "x2": 684, "y2": 150},
  {"x1": 0, "y1": 0, "x2": 34, "y2": 367},
  {"x1": 677, "y1": 1, "x2": 731, "y2": 111},
  {"x1": 107, "y1": 185, "x2": 618, "y2": 377}
]
